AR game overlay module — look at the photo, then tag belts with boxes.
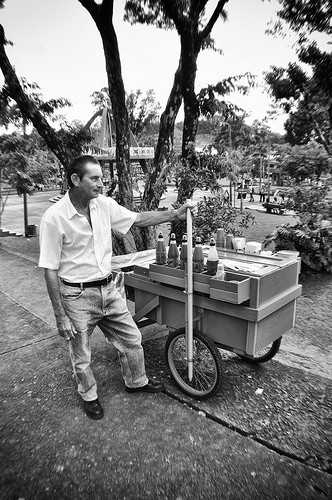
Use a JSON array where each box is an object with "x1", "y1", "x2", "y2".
[{"x1": 60, "y1": 272, "x2": 112, "y2": 288}]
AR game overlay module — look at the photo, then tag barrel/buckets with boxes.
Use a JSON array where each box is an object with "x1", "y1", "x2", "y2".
[{"x1": 278, "y1": 250, "x2": 299, "y2": 260}]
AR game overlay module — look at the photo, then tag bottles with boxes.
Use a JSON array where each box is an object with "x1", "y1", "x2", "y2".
[
  {"x1": 167, "y1": 233, "x2": 178, "y2": 268},
  {"x1": 156, "y1": 232, "x2": 166, "y2": 265},
  {"x1": 192, "y1": 237, "x2": 204, "y2": 273},
  {"x1": 206, "y1": 238, "x2": 219, "y2": 274},
  {"x1": 216, "y1": 264, "x2": 224, "y2": 280},
  {"x1": 179, "y1": 235, "x2": 187, "y2": 270}
]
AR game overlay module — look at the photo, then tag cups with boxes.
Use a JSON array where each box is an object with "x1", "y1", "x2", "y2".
[{"x1": 216, "y1": 228, "x2": 233, "y2": 250}]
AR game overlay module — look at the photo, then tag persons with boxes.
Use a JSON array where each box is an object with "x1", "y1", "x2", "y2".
[
  {"x1": 259, "y1": 186, "x2": 265, "y2": 202},
  {"x1": 266, "y1": 194, "x2": 291, "y2": 214},
  {"x1": 249, "y1": 186, "x2": 254, "y2": 202},
  {"x1": 38, "y1": 155, "x2": 198, "y2": 421}
]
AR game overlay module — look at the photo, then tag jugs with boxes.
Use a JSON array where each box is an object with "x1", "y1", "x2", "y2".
[
  {"x1": 246, "y1": 241, "x2": 263, "y2": 253},
  {"x1": 232, "y1": 237, "x2": 248, "y2": 251}
]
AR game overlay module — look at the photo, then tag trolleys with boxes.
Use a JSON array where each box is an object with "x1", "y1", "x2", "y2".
[{"x1": 112, "y1": 244, "x2": 304, "y2": 401}]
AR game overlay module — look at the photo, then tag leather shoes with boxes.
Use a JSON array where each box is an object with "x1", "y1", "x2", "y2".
[
  {"x1": 84, "y1": 398, "x2": 104, "y2": 419},
  {"x1": 126, "y1": 380, "x2": 165, "y2": 393}
]
